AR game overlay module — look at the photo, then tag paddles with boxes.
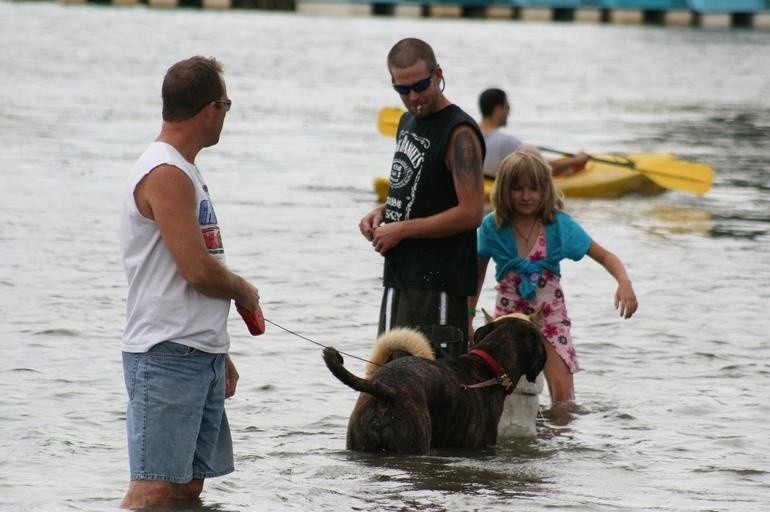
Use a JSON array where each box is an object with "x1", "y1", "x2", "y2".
[{"x1": 378, "y1": 106, "x2": 714, "y2": 197}]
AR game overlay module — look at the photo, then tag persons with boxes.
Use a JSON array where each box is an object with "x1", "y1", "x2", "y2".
[
  {"x1": 466, "y1": 144, "x2": 638, "y2": 413},
  {"x1": 358, "y1": 36, "x2": 487, "y2": 357},
  {"x1": 453, "y1": 130, "x2": 482, "y2": 195},
  {"x1": 477, "y1": 88, "x2": 592, "y2": 180},
  {"x1": 119, "y1": 53, "x2": 262, "y2": 510}
]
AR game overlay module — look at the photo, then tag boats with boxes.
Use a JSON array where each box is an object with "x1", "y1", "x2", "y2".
[{"x1": 370, "y1": 150, "x2": 679, "y2": 206}]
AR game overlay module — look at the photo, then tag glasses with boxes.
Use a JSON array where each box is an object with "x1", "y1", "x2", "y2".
[
  {"x1": 393, "y1": 66, "x2": 434, "y2": 95},
  {"x1": 217, "y1": 99, "x2": 231, "y2": 110}
]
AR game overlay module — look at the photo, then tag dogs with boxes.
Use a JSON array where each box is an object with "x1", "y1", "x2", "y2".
[
  {"x1": 323, "y1": 317, "x2": 548, "y2": 456},
  {"x1": 365, "y1": 301, "x2": 550, "y2": 439}
]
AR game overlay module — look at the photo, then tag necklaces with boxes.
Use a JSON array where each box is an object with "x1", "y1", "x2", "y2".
[{"x1": 511, "y1": 215, "x2": 541, "y2": 250}]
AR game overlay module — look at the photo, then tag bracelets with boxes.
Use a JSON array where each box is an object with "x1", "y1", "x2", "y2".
[{"x1": 467, "y1": 306, "x2": 475, "y2": 316}]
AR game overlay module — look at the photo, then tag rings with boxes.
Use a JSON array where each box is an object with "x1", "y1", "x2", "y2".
[{"x1": 373, "y1": 238, "x2": 377, "y2": 244}]
